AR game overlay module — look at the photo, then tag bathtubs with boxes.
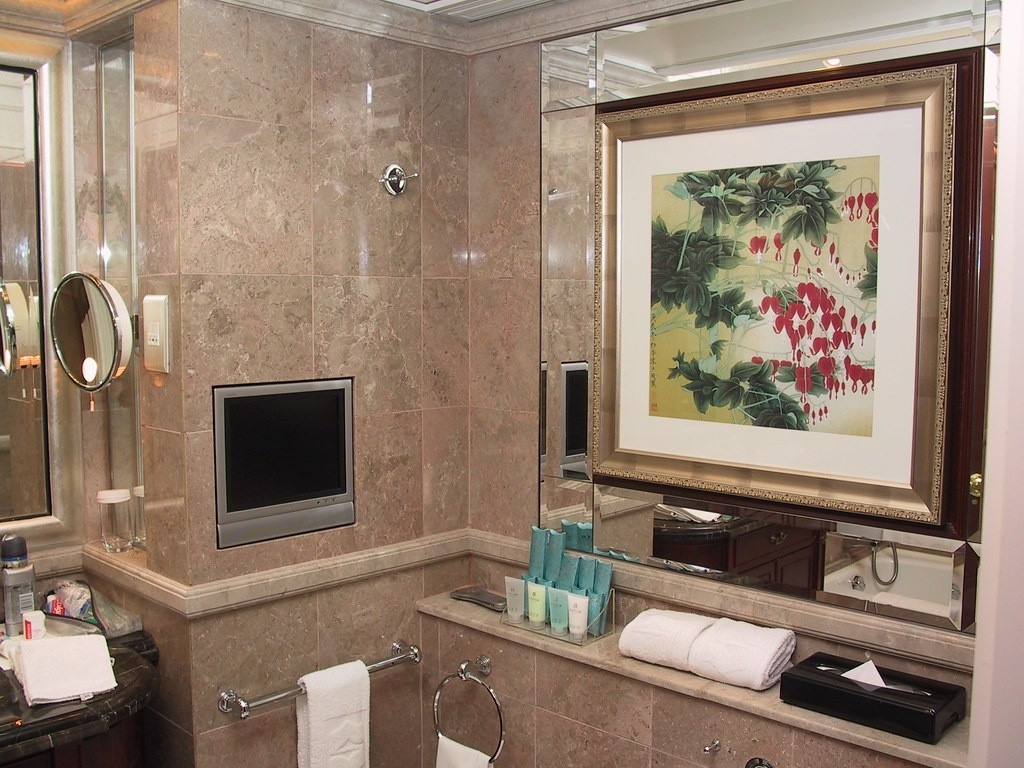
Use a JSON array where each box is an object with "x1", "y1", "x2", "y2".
[{"x1": 823, "y1": 545, "x2": 982, "y2": 620}]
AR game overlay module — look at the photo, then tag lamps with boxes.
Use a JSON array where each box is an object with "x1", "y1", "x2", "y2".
[{"x1": 82, "y1": 357, "x2": 97, "y2": 382}]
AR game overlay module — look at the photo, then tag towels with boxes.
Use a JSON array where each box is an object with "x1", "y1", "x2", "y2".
[
  {"x1": 435, "y1": 735, "x2": 494, "y2": 768},
  {"x1": 617, "y1": 607, "x2": 797, "y2": 692},
  {"x1": 294, "y1": 658, "x2": 371, "y2": 768},
  {"x1": 8, "y1": 634, "x2": 119, "y2": 708}
]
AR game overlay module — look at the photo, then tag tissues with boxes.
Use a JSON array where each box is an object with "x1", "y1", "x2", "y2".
[{"x1": 779, "y1": 651, "x2": 967, "y2": 746}]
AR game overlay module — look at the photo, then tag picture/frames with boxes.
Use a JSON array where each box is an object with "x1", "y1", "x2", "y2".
[{"x1": 592, "y1": 63, "x2": 957, "y2": 525}]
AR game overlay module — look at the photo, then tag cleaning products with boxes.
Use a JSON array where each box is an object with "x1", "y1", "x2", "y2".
[{"x1": 0, "y1": 530, "x2": 143, "y2": 641}]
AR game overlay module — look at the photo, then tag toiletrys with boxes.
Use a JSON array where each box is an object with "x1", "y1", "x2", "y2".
[
  {"x1": 559, "y1": 518, "x2": 593, "y2": 554},
  {"x1": 503, "y1": 526, "x2": 615, "y2": 643}
]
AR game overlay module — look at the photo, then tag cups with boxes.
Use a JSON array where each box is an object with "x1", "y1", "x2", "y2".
[{"x1": 97, "y1": 487, "x2": 137, "y2": 554}]
